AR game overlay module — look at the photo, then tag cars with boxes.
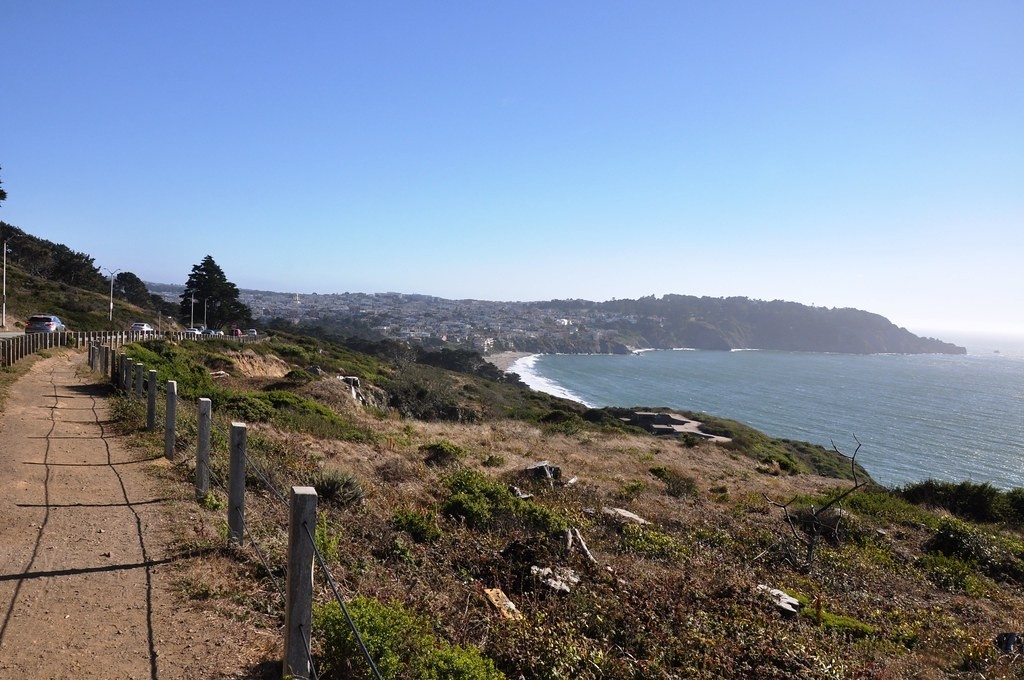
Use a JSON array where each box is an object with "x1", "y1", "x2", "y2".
[
  {"x1": 230, "y1": 329, "x2": 242, "y2": 337},
  {"x1": 202, "y1": 330, "x2": 214, "y2": 335},
  {"x1": 25, "y1": 315, "x2": 65, "y2": 335},
  {"x1": 248, "y1": 329, "x2": 257, "y2": 336},
  {"x1": 216, "y1": 331, "x2": 225, "y2": 335},
  {"x1": 186, "y1": 328, "x2": 201, "y2": 337},
  {"x1": 130, "y1": 323, "x2": 153, "y2": 338}
]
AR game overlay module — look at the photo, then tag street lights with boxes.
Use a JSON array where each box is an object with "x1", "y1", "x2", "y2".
[
  {"x1": 104, "y1": 268, "x2": 121, "y2": 321},
  {"x1": 1, "y1": 234, "x2": 22, "y2": 327},
  {"x1": 205, "y1": 296, "x2": 212, "y2": 330},
  {"x1": 191, "y1": 290, "x2": 200, "y2": 328}
]
